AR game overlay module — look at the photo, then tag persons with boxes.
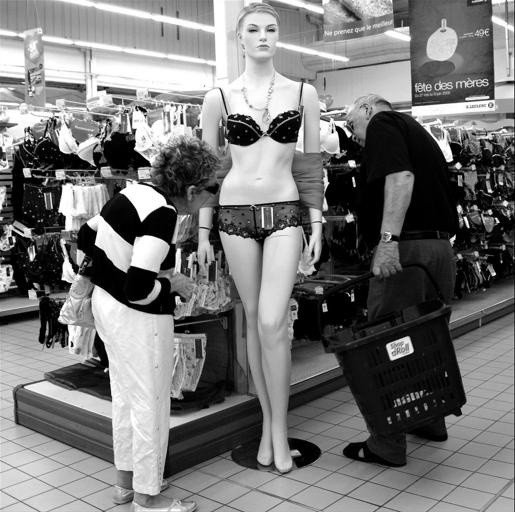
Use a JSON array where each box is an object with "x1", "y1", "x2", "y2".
[
  {"x1": 342, "y1": 93, "x2": 459, "y2": 467},
  {"x1": 77, "y1": 138, "x2": 223, "y2": 512},
  {"x1": 194, "y1": 2, "x2": 323, "y2": 473}
]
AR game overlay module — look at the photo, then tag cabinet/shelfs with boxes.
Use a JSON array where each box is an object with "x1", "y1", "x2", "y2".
[{"x1": 0, "y1": 92, "x2": 515, "y2": 479}]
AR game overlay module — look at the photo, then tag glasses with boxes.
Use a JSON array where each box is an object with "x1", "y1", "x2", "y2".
[
  {"x1": 202, "y1": 183, "x2": 219, "y2": 195},
  {"x1": 345, "y1": 124, "x2": 355, "y2": 133}
]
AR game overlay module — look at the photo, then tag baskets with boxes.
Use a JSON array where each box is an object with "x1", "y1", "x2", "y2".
[{"x1": 316, "y1": 262, "x2": 465, "y2": 436}]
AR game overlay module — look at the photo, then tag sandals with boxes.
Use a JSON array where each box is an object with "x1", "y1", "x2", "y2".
[
  {"x1": 342, "y1": 441, "x2": 407, "y2": 467},
  {"x1": 408, "y1": 428, "x2": 448, "y2": 441}
]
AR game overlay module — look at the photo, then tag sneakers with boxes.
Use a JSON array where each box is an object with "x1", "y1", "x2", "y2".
[
  {"x1": 131, "y1": 497, "x2": 198, "y2": 512},
  {"x1": 112, "y1": 479, "x2": 169, "y2": 504}
]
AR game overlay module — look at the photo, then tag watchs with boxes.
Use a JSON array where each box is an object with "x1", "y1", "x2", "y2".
[{"x1": 379, "y1": 232, "x2": 402, "y2": 246}]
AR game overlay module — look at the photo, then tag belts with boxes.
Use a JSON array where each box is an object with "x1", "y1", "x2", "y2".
[{"x1": 398, "y1": 231, "x2": 453, "y2": 241}]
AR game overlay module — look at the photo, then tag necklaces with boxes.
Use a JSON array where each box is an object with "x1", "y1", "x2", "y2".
[{"x1": 238, "y1": 71, "x2": 277, "y2": 124}]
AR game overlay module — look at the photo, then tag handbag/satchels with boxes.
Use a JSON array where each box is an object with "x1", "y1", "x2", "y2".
[{"x1": 58, "y1": 274, "x2": 94, "y2": 328}]
